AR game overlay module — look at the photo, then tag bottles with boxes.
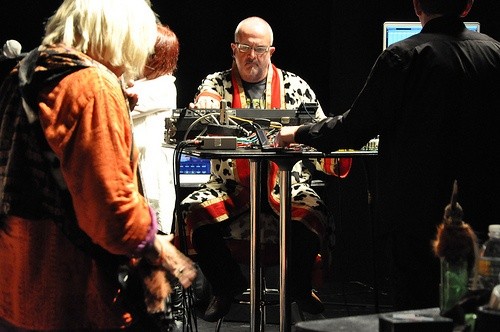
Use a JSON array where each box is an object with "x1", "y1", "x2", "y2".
[{"x1": 476, "y1": 224, "x2": 500, "y2": 290}]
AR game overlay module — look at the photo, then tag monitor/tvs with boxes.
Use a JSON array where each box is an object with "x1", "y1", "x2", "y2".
[{"x1": 383, "y1": 22, "x2": 481, "y2": 53}]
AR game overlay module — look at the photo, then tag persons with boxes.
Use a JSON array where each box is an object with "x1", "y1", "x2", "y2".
[
  {"x1": 175, "y1": 16, "x2": 327, "y2": 322},
  {"x1": 126, "y1": 25, "x2": 177, "y2": 235},
  {"x1": 274, "y1": 0, "x2": 500, "y2": 307},
  {"x1": 0, "y1": 0, "x2": 196, "y2": 332}
]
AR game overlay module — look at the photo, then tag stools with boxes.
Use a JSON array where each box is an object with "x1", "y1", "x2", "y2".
[{"x1": 214, "y1": 239, "x2": 324, "y2": 332}]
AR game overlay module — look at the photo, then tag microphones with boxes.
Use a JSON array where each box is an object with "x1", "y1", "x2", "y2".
[{"x1": 0, "y1": 39, "x2": 22, "y2": 64}]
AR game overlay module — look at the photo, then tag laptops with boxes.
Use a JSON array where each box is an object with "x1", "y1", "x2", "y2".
[{"x1": 178, "y1": 155, "x2": 211, "y2": 188}]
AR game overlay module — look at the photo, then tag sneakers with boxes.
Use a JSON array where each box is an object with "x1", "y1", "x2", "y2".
[
  {"x1": 162, "y1": 259, "x2": 228, "y2": 331},
  {"x1": 203, "y1": 288, "x2": 229, "y2": 320},
  {"x1": 286, "y1": 258, "x2": 324, "y2": 314}
]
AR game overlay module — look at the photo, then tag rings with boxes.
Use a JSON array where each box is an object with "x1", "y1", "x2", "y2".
[{"x1": 179, "y1": 266, "x2": 184, "y2": 273}]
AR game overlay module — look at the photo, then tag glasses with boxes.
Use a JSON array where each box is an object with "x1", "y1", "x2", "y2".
[{"x1": 234, "y1": 43, "x2": 272, "y2": 56}]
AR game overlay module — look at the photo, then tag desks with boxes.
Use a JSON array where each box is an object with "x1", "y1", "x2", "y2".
[{"x1": 165, "y1": 142, "x2": 379, "y2": 332}]
